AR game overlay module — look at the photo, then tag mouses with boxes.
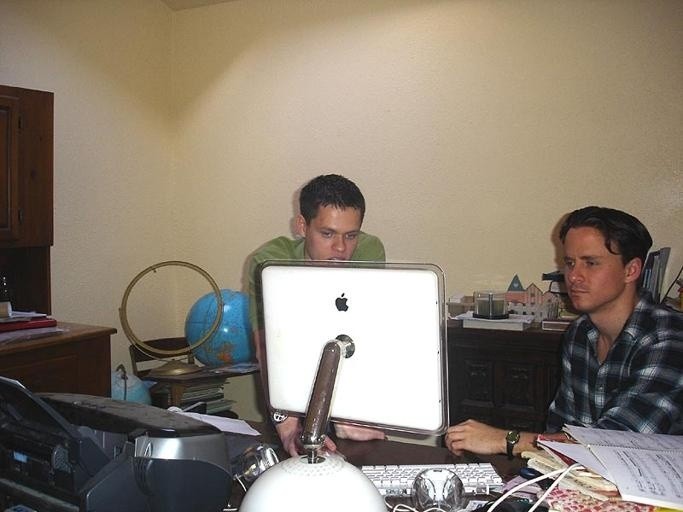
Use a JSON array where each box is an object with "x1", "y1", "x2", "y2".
[{"x1": 322, "y1": 447, "x2": 347, "y2": 461}]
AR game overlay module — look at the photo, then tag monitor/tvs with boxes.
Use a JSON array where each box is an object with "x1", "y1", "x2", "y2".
[{"x1": 253, "y1": 261, "x2": 449, "y2": 436}]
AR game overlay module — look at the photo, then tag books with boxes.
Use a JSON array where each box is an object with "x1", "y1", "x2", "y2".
[
  {"x1": 519, "y1": 423, "x2": 682, "y2": 512},
  {"x1": 460, "y1": 312, "x2": 533, "y2": 332},
  {"x1": 0, "y1": 317, "x2": 59, "y2": 336},
  {"x1": 173, "y1": 406, "x2": 262, "y2": 438},
  {"x1": 0, "y1": 324, "x2": 63, "y2": 345},
  {"x1": 0, "y1": 310, "x2": 47, "y2": 323}
]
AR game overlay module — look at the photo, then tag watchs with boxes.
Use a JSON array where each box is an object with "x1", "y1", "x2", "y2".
[
  {"x1": 268, "y1": 409, "x2": 289, "y2": 427},
  {"x1": 504, "y1": 428, "x2": 525, "y2": 458}
]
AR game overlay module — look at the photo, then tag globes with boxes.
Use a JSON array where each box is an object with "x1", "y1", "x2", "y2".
[
  {"x1": 184, "y1": 289, "x2": 258, "y2": 366},
  {"x1": 111, "y1": 363, "x2": 153, "y2": 406}
]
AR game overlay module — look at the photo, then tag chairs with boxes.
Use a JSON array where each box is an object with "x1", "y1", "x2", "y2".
[{"x1": 127, "y1": 336, "x2": 195, "y2": 385}]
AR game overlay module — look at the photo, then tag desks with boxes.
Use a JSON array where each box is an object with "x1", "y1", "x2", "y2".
[
  {"x1": 0, "y1": 84, "x2": 119, "y2": 400},
  {"x1": 225, "y1": 416, "x2": 544, "y2": 511}
]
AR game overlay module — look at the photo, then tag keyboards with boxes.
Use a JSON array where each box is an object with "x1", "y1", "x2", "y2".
[{"x1": 360, "y1": 462, "x2": 503, "y2": 497}]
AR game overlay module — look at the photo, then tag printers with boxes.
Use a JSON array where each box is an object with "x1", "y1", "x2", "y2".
[{"x1": 0, "y1": 377, "x2": 232, "y2": 512}]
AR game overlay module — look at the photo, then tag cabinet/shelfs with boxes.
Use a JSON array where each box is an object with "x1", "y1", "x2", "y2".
[{"x1": 445, "y1": 321, "x2": 564, "y2": 430}]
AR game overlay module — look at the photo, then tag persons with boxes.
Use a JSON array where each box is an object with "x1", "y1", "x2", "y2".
[
  {"x1": 440, "y1": 204, "x2": 682, "y2": 460},
  {"x1": 244, "y1": 170, "x2": 393, "y2": 461}
]
